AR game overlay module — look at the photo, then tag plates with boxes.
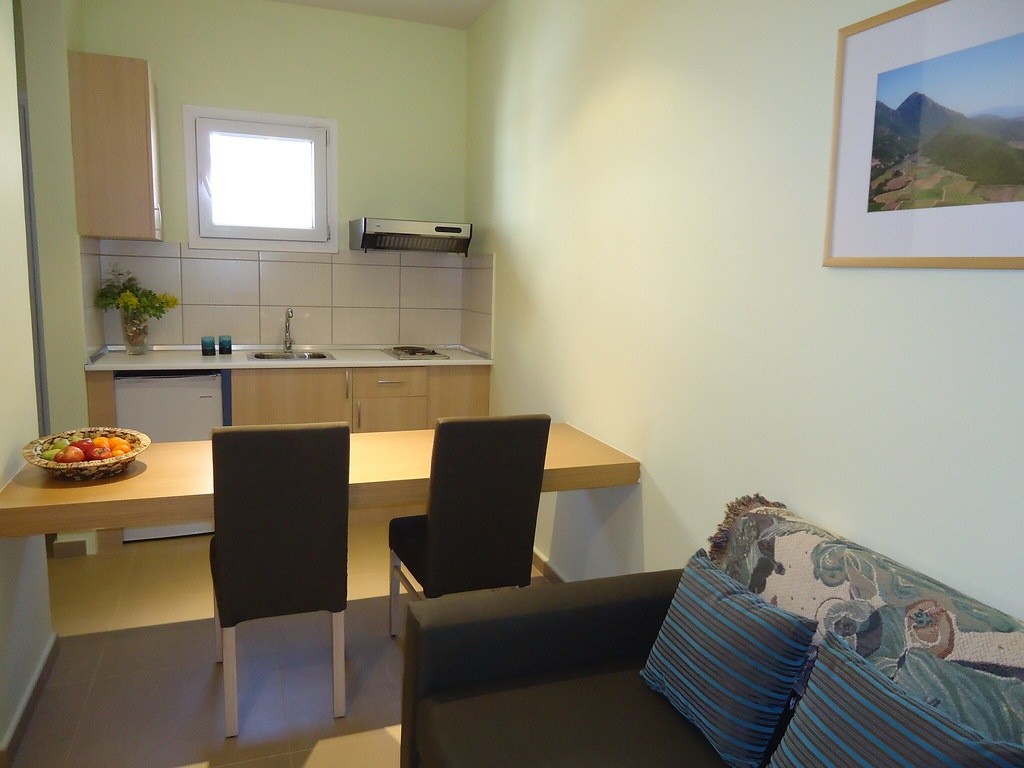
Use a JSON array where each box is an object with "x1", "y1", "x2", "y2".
[{"x1": 22, "y1": 426, "x2": 151, "y2": 481}]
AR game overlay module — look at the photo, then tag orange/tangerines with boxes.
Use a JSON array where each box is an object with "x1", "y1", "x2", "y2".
[{"x1": 93, "y1": 436, "x2": 132, "y2": 456}]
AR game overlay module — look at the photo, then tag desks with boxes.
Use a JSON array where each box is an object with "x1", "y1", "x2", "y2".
[{"x1": 0, "y1": 422, "x2": 642, "y2": 541}]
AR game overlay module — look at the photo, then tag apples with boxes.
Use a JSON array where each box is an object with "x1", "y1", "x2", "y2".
[{"x1": 55, "y1": 439, "x2": 112, "y2": 463}]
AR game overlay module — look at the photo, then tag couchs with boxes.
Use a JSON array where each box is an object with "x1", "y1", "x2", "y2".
[{"x1": 400, "y1": 493, "x2": 1024, "y2": 768}]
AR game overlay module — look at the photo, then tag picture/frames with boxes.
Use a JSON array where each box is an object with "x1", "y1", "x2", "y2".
[{"x1": 821, "y1": 0, "x2": 1024, "y2": 270}]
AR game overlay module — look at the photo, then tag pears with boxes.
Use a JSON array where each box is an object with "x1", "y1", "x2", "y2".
[{"x1": 39, "y1": 435, "x2": 82, "y2": 461}]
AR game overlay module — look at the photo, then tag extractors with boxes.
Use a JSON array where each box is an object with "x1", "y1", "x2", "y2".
[{"x1": 349, "y1": 217, "x2": 474, "y2": 259}]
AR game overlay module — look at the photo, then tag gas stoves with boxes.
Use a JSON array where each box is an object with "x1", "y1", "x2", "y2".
[{"x1": 381, "y1": 344, "x2": 450, "y2": 362}]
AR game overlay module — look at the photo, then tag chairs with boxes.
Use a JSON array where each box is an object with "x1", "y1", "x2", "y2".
[
  {"x1": 390, "y1": 412, "x2": 552, "y2": 639},
  {"x1": 211, "y1": 420, "x2": 351, "y2": 739}
]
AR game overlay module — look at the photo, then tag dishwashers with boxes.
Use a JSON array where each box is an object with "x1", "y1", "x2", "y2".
[{"x1": 117, "y1": 374, "x2": 224, "y2": 443}]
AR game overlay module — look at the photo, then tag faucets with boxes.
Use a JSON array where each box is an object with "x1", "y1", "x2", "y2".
[{"x1": 285, "y1": 308, "x2": 293, "y2": 353}]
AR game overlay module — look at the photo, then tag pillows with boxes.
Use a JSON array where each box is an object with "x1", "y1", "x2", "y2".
[
  {"x1": 765, "y1": 627, "x2": 1024, "y2": 768},
  {"x1": 637, "y1": 545, "x2": 819, "y2": 768}
]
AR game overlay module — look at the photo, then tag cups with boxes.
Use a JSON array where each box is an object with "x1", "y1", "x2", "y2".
[
  {"x1": 201, "y1": 336, "x2": 216, "y2": 356},
  {"x1": 219, "y1": 335, "x2": 232, "y2": 354}
]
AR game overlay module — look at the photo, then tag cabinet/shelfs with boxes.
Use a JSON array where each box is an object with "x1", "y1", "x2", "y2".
[
  {"x1": 353, "y1": 366, "x2": 428, "y2": 434},
  {"x1": 427, "y1": 366, "x2": 490, "y2": 430},
  {"x1": 67, "y1": 49, "x2": 163, "y2": 243},
  {"x1": 232, "y1": 367, "x2": 353, "y2": 433}
]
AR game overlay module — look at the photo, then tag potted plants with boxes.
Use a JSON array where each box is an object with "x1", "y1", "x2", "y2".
[{"x1": 91, "y1": 259, "x2": 179, "y2": 355}]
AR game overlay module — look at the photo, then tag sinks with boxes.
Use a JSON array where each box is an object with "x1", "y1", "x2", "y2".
[{"x1": 246, "y1": 351, "x2": 336, "y2": 361}]
